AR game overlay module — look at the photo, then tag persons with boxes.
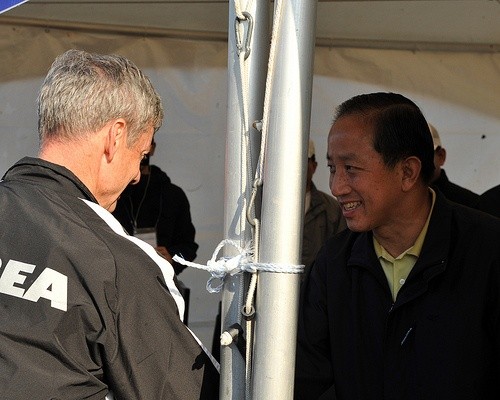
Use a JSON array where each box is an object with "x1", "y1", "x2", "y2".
[
  {"x1": 0, "y1": 50, "x2": 224, "y2": 400},
  {"x1": 301, "y1": 126, "x2": 500, "y2": 292},
  {"x1": 293, "y1": 93, "x2": 500, "y2": 400},
  {"x1": 115, "y1": 139, "x2": 199, "y2": 278}
]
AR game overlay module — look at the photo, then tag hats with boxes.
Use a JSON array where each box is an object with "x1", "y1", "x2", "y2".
[
  {"x1": 306, "y1": 138, "x2": 316, "y2": 159},
  {"x1": 427, "y1": 123, "x2": 441, "y2": 151}
]
